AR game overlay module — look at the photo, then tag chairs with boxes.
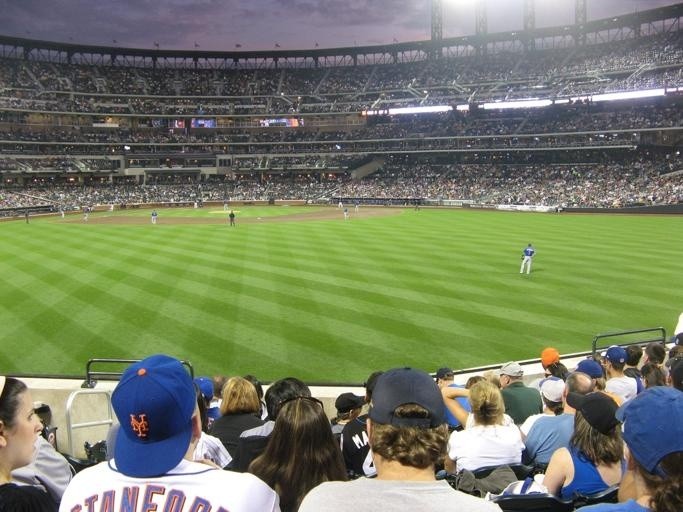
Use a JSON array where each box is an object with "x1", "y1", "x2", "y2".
[{"x1": 435, "y1": 457, "x2": 620, "y2": 512}]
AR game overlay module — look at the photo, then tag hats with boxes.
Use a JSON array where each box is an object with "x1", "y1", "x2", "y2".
[
  {"x1": 109, "y1": 353, "x2": 197, "y2": 478},
  {"x1": 566, "y1": 391, "x2": 621, "y2": 435},
  {"x1": 334, "y1": 392, "x2": 367, "y2": 413},
  {"x1": 367, "y1": 366, "x2": 448, "y2": 428},
  {"x1": 435, "y1": 367, "x2": 454, "y2": 384},
  {"x1": 539, "y1": 377, "x2": 566, "y2": 403},
  {"x1": 600, "y1": 345, "x2": 628, "y2": 364},
  {"x1": 575, "y1": 359, "x2": 603, "y2": 378},
  {"x1": 495, "y1": 361, "x2": 524, "y2": 377},
  {"x1": 613, "y1": 385, "x2": 683, "y2": 480},
  {"x1": 193, "y1": 376, "x2": 214, "y2": 401}
]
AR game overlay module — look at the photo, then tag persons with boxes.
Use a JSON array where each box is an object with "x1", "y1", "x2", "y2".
[{"x1": 520, "y1": 242, "x2": 537, "y2": 274}]
